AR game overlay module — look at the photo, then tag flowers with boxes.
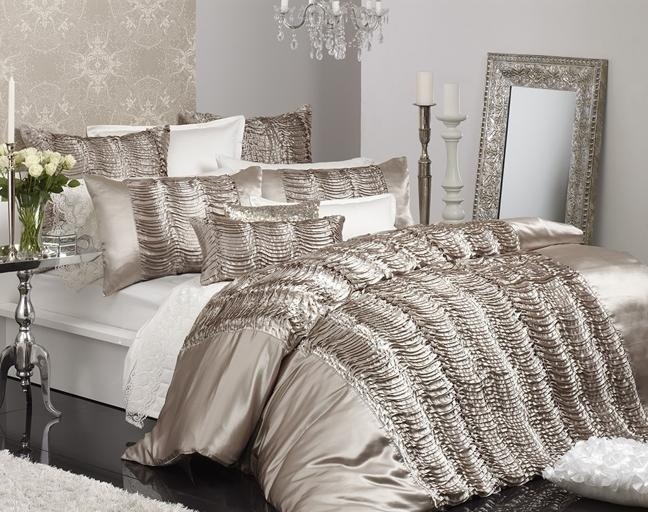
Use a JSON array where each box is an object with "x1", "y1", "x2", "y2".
[{"x1": 0, "y1": 139, "x2": 80, "y2": 251}]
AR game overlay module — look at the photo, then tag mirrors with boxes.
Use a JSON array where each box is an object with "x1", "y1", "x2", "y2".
[{"x1": 469, "y1": 49, "x2": 608, "y2": 244}]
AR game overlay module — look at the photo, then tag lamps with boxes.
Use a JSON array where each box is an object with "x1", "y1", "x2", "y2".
[{"x1": 269, "y1": 0, "x2": 390, "y2": 61}]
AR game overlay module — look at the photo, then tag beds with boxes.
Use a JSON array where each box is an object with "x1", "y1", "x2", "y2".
[{"x1": 0, "y1": 217, "x2": 647, "y2": 511}]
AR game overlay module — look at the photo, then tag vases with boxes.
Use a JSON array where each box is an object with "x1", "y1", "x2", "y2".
[{"x1": 13, "y1": 192, "x2": 46, "y2": 259}]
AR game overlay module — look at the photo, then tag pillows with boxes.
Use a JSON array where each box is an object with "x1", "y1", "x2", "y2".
[
  {"x1": 539, "y1": 431, "x2": 647, "y2": 503},
  {"x1": 21, "y1": 103, "x2": 413, "y2": 287}
]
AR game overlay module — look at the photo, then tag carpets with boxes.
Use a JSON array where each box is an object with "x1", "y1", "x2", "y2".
[{"x1": 0, "y1": 448, "x2": 198, "y2": 512}]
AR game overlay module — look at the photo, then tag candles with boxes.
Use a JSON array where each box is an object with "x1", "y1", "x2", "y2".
[
  {"x1": 414, "y1": 69, "x2": 433, "y2": 105},
  {"x1": 5, "y1": 77, "x2": 18, "y2": 144},
  {"x1": 440, "y1": 81, "x2": 460, "y2": 118}
]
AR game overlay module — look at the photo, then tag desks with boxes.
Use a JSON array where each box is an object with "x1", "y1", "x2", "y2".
[{"x1": 0, "y1": 237, "x2": 104, "y2": 420}]
